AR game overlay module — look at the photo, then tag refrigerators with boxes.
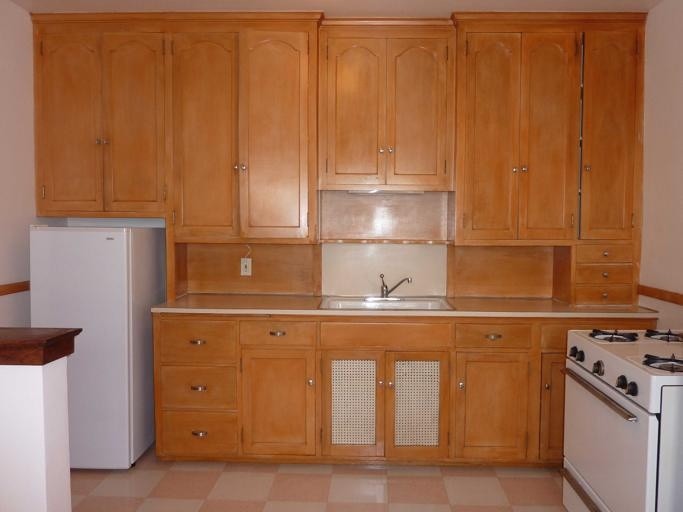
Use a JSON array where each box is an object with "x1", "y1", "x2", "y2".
[{"x1": 28, "y1": 216, "x2": 166, "y2": 470}]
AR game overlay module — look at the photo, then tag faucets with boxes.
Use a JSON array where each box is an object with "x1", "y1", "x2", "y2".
[{"x1": 380, "y1": 274, "x2": 412, "y2": 297}]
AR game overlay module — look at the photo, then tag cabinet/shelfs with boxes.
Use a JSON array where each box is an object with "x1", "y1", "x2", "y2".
[
  {"x1": 317, "y1": 19, "x2": 454, "y2": 246},
  {"x1": 447, "y1": 11, "x2": 648, "y2": 311},
  {"x1": 153, "y1": 313, "x2": 318, "y2": 465},
  {"x1": 30, "y1": 11, "x2": 165, "y2": 218},
  {"x1": 165, "y1": 13, "x2": 320, "y2": 304},
  {"x1": 450, "y1": 319, "x2": 658, "y2": 468},
  {"x1": 316, "y1": 317, "x2": 454, "y2": 467}
]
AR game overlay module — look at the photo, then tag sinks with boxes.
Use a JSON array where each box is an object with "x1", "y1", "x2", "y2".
[{"x1": 319, "y1": 297, "x2": 453, "y2": 310}]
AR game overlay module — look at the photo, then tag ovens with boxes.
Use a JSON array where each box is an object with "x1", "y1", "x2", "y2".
[{"x1": 562, "y1": 331, "x2": 683, "y2": 512}]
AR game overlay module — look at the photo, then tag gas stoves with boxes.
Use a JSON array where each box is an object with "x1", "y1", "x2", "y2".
[{"x1": 566, "y1": 329, "x2": 683, "y2": 416}]
